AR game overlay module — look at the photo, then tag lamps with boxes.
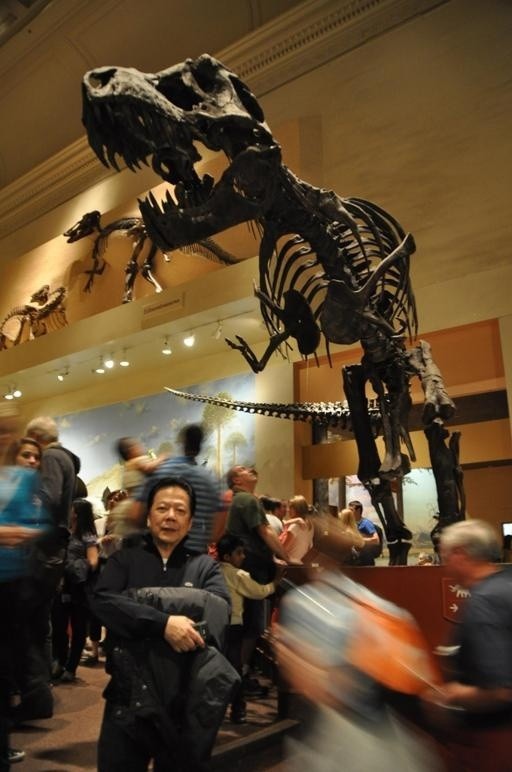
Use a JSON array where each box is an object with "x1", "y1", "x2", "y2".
[{"x1": 0, "y1": 307, "x2": 258, "y2": 402}]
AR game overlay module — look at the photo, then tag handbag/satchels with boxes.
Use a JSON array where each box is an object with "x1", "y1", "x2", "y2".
[
  {"x1": 31, "y1": 558, "x2": 66, "y2": 590},
  {"x1": 370, "y1": 525, "x2": 382, "y2": 558}
]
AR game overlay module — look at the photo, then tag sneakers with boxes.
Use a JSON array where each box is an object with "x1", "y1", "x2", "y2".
[
  {"x1": 243, "y1": 679, "x2": 268, "y2": 697},
  {"x1": 12, "y1": 639, "x2": 104, "y2": 719},
  {"x1": 230, "y1": 713, "x2": 246, "y2": 723},
  {"x1": 7, "y1": 750, "x2": 25, "y2": 762}
]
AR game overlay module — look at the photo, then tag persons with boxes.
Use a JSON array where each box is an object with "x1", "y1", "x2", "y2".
[{"x1": 0, "y1": 411, "x2": 511, "y2": 772}]
